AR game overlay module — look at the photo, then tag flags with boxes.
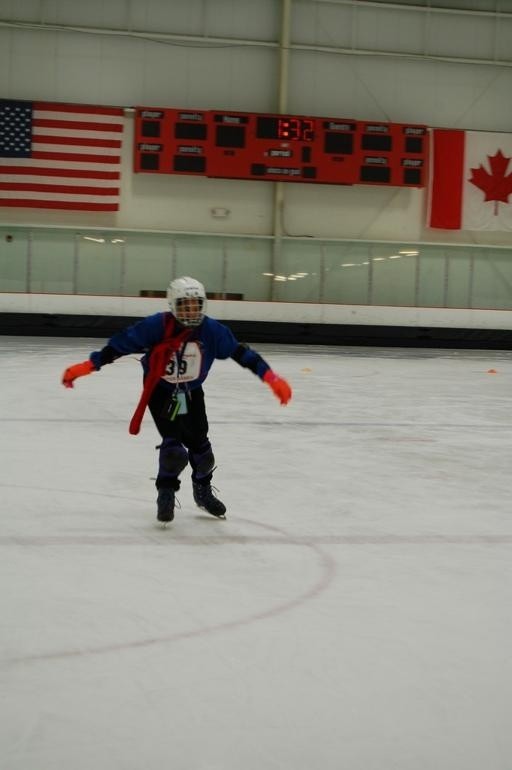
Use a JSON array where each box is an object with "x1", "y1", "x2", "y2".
[
  {"x1": 426, "y1": 128, "x2": 512, "y2": 231},
  {"x1": 0, "y1": 98, "x2": 123, "y2": 214}
]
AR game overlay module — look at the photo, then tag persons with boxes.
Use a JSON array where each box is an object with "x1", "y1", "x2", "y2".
[{"x1": 64, "y1": 274, "x2": 291, "y2": 529}]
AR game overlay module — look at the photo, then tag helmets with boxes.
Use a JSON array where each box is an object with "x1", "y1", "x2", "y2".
[{"x1": 166, "y1": 276, "x2": 208, "y2": 328}]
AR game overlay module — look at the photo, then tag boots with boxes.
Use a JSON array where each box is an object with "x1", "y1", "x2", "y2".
[
  {"x1": 190, "y1": 476, "x2": 227, "y2": 516},
  {"x1": 153, "y1": 474, "x2": 181, "y2": 523}
]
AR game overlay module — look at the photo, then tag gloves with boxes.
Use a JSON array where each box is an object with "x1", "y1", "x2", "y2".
[
  {"x1": 62, "y1": 360, "x2": 94, "y2": 389},
  {"x1": 261, "y1": 368, "x2": 292, "y2": 406}
]
